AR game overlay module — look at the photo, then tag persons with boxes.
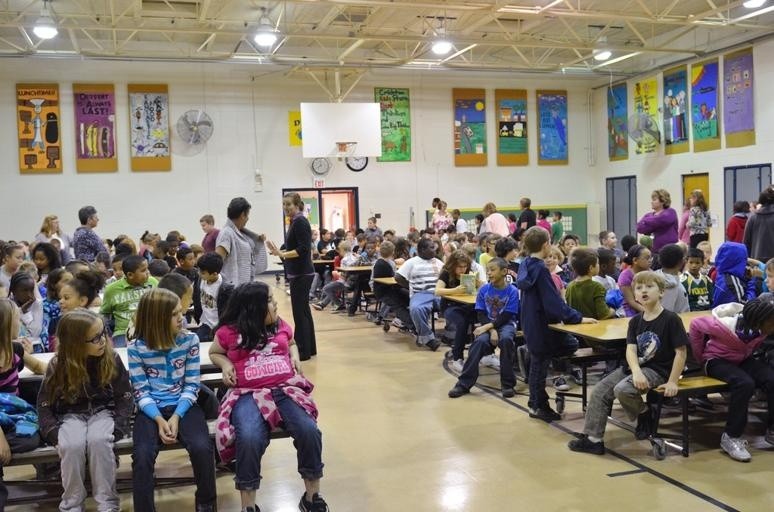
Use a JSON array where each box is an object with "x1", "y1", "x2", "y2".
[
  {"x1": 265, "y1": 192, "x2": 317, "y2": 361},
  {"x1": 215, "y1": 196, "x2": 270, "y2": 285},
  {"x1": 1, "y1": 202, "x2": 330, "y2": 511},
  {"x1": 740, "y1": 187, "x2": 772, "y2": 263},
  {"x1": 675, "y1": 200, "x2": 693, "y2": 247},
  {"x1": 683, "y1": 190, "x2": 713, "y2": 249},
  {"x1": 634, "y1": 187, "x2": 678, "y2": 270},
  {"x1": 329, "y1": 197, "x2": 774, "y2": 461}
]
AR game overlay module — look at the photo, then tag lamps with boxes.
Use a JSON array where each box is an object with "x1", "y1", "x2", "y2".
[
  {"x1": 593, "y1": 26, "x2": 611, "y2": 61},
  {"x1": 32, "y1": 0, "x2": 57, "y2": 39},
  {"x1": 254, "y1": 5, "x2": 276, "y2": 47},
  {"x1": 431, "y1": 14, "x2": 451, "y2": 55}
]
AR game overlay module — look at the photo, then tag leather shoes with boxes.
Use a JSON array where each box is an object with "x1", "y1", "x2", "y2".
[{"x1": 689, "y1": 395, "x2": 714, "y2": 408}]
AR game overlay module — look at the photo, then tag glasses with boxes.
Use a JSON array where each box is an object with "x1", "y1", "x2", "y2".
[{"x1": 85, "y1": 327, "x2": 106, "y2": 345}]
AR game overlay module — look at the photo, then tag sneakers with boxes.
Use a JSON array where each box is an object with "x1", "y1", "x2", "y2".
[
  {"x1": 434, "y1": 311, "x2": 440, "y2": 320},
  {"x1": 449, "y1": 381, "x2": 470, "y2": 397},
  {"x1": 297, "y1": 491, "x2": 330, "y2": 512},
  {"x1": 634, "y1": 402, "x2": 657, "y2": 440},
  {"x1": 478, "y1": 353, "x2": 501, "y2": 371},
  {"x1": 764, "y1": 428, "x2": 774, "y2": 445},
  {"x1": 426, "y1": 339, "x2": 440, "y2": 352},
  {"x1": 391, "y1": 316, "x2": 403, "y2": 329},
  {"x1": 528, "y1": 403, "x2": 561, "y2": 421},
  {"x1": 308, "y1": 297, "x2": 358, "y2": 317},
  {"x1": 568, "y1": 437, "x2": 604, "y2": 455},
  {"x1": 441, "y1": 335, "x2": 456, "y2": 347},
  {"x1": 448, "y1": 358, "x2": 465, "y2": 377},
  {"x1": 551, "y1": 375, "x2": 570, "y2": 391},
  {"x1": 720, "y1": 433, "x2": 752, "y2": 461},
  {"x1": 570, "y1": 367, "x2": 583, "y2": 385},
  {"x1": 501, "y1": 384, "x2": 514, "y2": 398}
]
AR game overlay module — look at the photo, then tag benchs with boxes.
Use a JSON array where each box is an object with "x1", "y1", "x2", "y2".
[{"x1": 4, "y1": 373, "x2": 256, "y2": 506}]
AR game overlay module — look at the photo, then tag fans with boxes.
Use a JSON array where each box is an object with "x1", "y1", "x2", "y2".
[
  {"x1": 626, "y1": 112, "x2": 660, "y2": 145},
  {"x1": 176, "y1": 108, "x2": 213, "y2": 146}
]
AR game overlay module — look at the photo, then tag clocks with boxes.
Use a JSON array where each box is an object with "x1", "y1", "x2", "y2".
[
  {"x1": 312, "y1": 157, "x2": 328, "y2": 175},
  {"x1": 344, "y1": 157, "x2": 368, "y2": 172}
]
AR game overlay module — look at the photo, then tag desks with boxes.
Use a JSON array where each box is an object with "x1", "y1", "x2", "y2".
[{"x1": 16, "y1": 339, "x2": 222, "y2": 401}]
